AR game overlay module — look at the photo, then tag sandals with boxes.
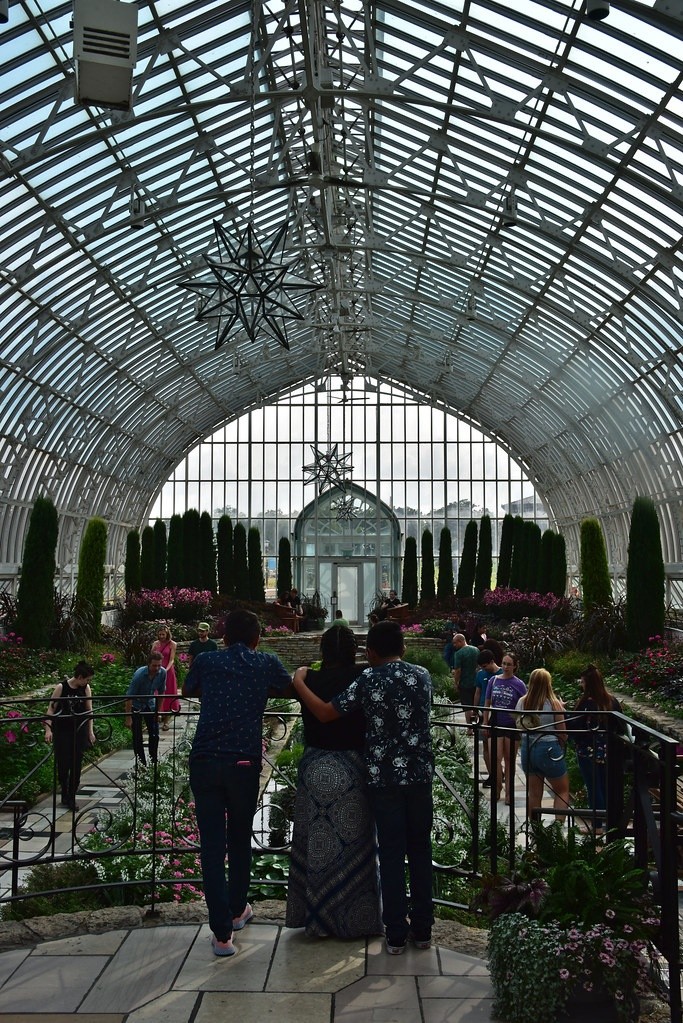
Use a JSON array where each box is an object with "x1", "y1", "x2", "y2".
[{"x1": 162, "y1": 723, "x2": 168, "y2": 730}]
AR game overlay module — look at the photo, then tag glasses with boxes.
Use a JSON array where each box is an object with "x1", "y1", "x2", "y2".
[{"x1": 502, "y1": 661, "x2": 514, "y2": 667}]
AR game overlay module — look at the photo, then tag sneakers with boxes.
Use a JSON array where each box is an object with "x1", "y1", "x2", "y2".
[
  {"x1": 212, "y1": 928, "x2": 234, "y2": 956},
  {"x1": 231, "y1": 902, "x2": 253, "y2": 929}
]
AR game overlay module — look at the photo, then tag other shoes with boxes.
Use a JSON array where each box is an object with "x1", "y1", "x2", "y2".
[
  {"x1": 61, "y1": 787, "x2": 68, "y2": 804},
  {"x1": 385, "y1": 932, "x2": 407, "y2": 954},
  {"x1": 577, "y1": 826, "x2": 603, "y2": 834},
  {"x1": 68, "y1": 799, "x2": 79, "y2": 810},
  {"x1": 483, "y1": 771, "x2": 504, "y2": 787},
  {"x1": 408, "y1": 924, "x2": 430, "y2": 948}
]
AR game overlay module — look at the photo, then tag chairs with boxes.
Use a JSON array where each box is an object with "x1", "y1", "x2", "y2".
[
  {"x1": 272, "y1": 599, "x2": 300, "y2": 632},
  {"x1": 381, "y1": 601, "x2": 410, "y2": 620}
]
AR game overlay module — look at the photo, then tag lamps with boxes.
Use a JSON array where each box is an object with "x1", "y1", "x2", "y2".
[
  {"x1": 124, "y1": 181, "x2": 146, "y2": 231},
  {"x1": 0, "y1": 0, "x2": 9, "y2": 24}
]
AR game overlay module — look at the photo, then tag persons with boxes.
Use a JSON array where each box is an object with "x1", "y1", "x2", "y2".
[
  {"x1": 43, "y1": 661, "x2": 95, "y2": 812},
  {"x1": 513, "y1": 667, "x2": 569, "y2": 830},
  {"x1": 292, "y1": 623, "x2": 438, "y2": 956},
  {"x1": 381, "y1": 588, "x2": 402, "y2": 620},
  {"x1": 293, "y1": 626, "x2": 385, "y2": 941},
  {"x1": 559, "y1": 663, "x2": 628, "y2": 835},
  {"x1": 329, "y1": 609, "x2": 349, "y2": 627},
  {"x1": 442, "y1": 612, "x2": 527, "y2": 805},
  {"x1": 151, "y1": 625, "x2": 179, "y2": 730},
  {"x1": 183, "y1": 609, "x2": 297, "y2": 959},
  {"x1": 122, "y1": 651, "x2": 166, "y2": 768},
  {"x1": 274, "y1": 588, "x2": 304, "y2": 619}
]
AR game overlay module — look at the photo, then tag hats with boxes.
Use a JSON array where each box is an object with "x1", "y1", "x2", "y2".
[{"x1": 198, "y1": 622, "x2": 209, "y2": 631}]
